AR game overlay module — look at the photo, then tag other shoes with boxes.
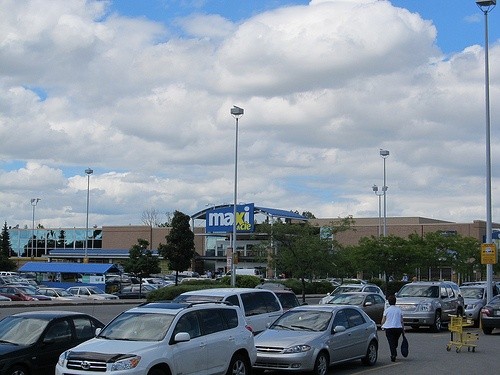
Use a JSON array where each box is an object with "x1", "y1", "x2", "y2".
[{"x1": 391, "y1": 355, "x2": 396, "y2": 362}]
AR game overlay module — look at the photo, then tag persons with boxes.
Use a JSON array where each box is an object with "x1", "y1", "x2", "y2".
[
  {"x1": 401, "y1": 273, "x2": 408, "y2": 282},
  {"x1": 388, "y1": 274, "x2": 395, "y2": 281},
  {"x1": 381, "y1": 295, "x2": 404, "y2": 363},
  {"x1": 280, "y1": 270, "x2": 285, "y2": 279},
  {"x1": 492, "y1": 281, "x2": 499, "y2": 297},
  {"x1": 411, "y1": 273, "x2": 418, "y2": 284},
  {"x1": 204, "y1": 267, "x2": 212, "y2": 279}
]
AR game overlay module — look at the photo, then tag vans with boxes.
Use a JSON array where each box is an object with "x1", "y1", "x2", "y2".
[{"x1": 395, "y1": 280, "x2": 465, "y2": 333}]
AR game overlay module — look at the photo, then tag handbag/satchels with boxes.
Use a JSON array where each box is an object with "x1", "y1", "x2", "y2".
[{"x1": 401, "y1": 333, "x2": 408, "y2": 357}]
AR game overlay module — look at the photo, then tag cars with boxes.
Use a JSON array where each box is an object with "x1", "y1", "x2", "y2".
[
  {"x1": 253, "y1": 304, "x2": 380, "y2": 375},
  {"x1": 0, "y1": 269, "x2": 366, "y2": 302},
  {"x1": 0, "y1": 311, "x2": 107, "y2": 375},
  {"x1": 271, "y1": 287, "x2": 301, "y2": 312},
  {"x1": 54, "y1": 300, "x2": 258, "y2": 375},
  {"x1": 172, "y1": 287, "x2": 285, "y2": 335},
  {"x1": 327, "y1": 292, "x2": 386, "y2": 323},
  {"x1": 459, "y1": 281, "x2": 500, "y2": 335},
  {"x1": 318, "y1": 284, "x2": 386, "y2": 305}
]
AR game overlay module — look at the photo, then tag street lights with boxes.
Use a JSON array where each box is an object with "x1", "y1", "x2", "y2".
[
  {"x1": 379, "y1": 149, "x2": 390, "y2": 237},
  {"x1": 82, "y1": 167, "x2": 94, "y2": 259},
  {"x1": 229, "y1": 106, "x2": 248, "y2": 290},
  {"x1": 30, "y1": 198, "x2": 40, "y2": 259},
  {"x1": 474, "y1": 0, "x2": 498, "y2": 304},
  {"x1": 373, "y1": 185, "x2": 389, "y2": 237}
]
